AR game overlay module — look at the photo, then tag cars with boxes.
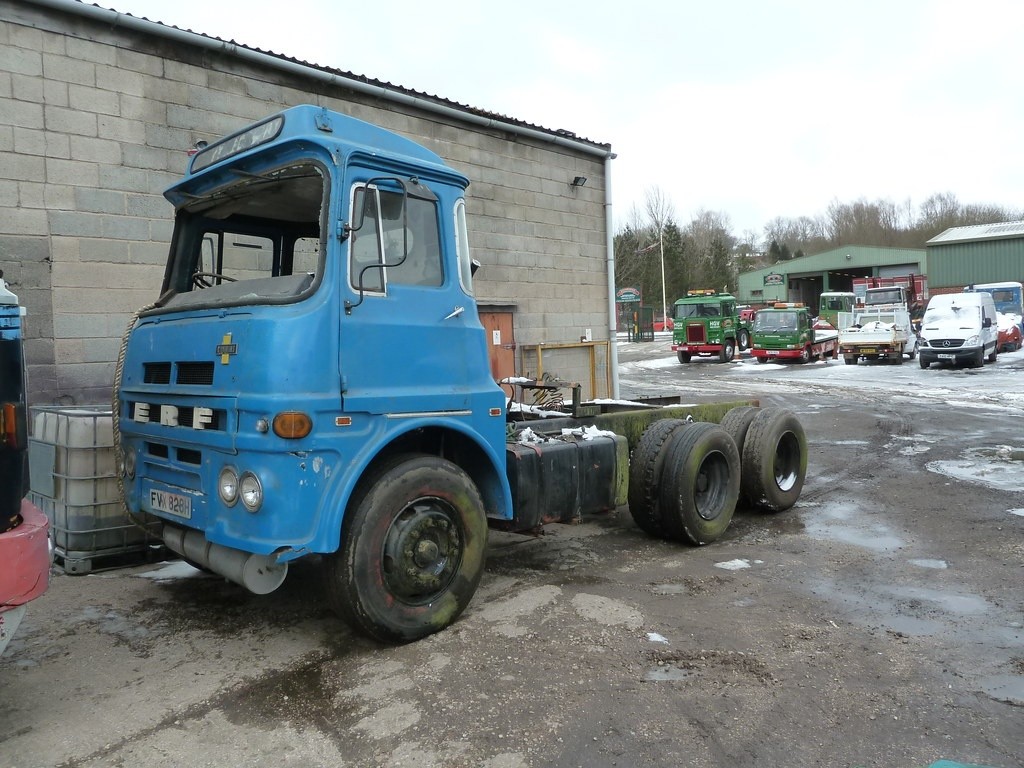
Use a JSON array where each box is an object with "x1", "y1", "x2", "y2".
[{"x1": 641, "y1": 316, "x2": 673, "y2": 331}]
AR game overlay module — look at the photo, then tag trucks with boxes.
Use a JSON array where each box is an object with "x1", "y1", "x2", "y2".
[
  {"x1": 863, "y1": 286, "x2": 924, "y2": 319},
  {"x1": 749, "y1": 303, "x2": 839, "y2": 364},
  {"x1": 963, "y1": 281, "x2": 1023, "y2": 354},
  {"x1": 837, "y1": 312, "x2": 920, "y2": 366},
  {"x1": 671, "y1": 289, "x2": 751, "y2": 363},
  {"x1": 0, "y1": 273, "x2": 55, "y2": 654}
]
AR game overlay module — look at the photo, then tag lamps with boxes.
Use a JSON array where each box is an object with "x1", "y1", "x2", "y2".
[
  {"x1": 846, "y1": 255, "x2": 851, "y2": 259},
  {"x1": 571, "y1": 177, "x2": 587, "y2": 186}
]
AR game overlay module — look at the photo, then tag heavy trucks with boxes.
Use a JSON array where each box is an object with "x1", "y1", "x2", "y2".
[{"x1": 114, "y1": 102, "x2": 808, "y2": 645}]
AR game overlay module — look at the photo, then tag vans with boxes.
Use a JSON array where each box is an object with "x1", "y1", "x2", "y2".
[{"x1": 916, "y1": 292, "x2": 998, "y2": 369}]
